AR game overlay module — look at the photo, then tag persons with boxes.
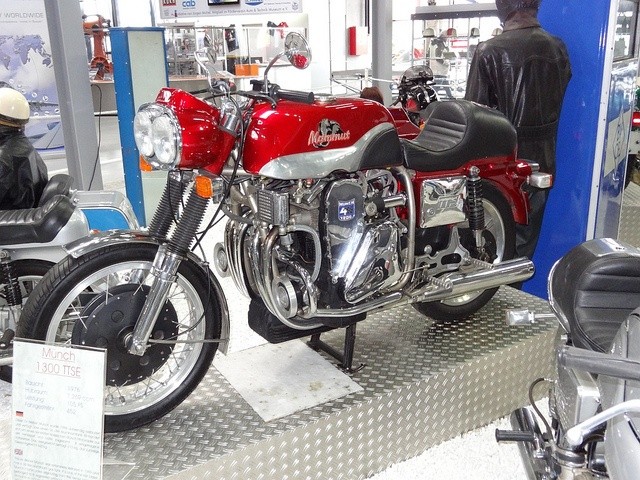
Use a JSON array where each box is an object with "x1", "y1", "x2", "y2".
[
  {"x1": 359, "y1": 85, "x2": 383, "y2": 105},
  {"x1": 463, "y1": 0, "x2": 572, "y2": 291},
  {"x1": 426, "y1": 30, "x2": 455, "y2": 99},
  {"x1": 0, "y1": 84, "x2": 50, "y2": 211}
]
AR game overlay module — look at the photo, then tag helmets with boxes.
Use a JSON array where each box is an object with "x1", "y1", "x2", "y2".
[
  {"x1": 495, "y1": 0, "x2": 540, "y2": 26},
  {"x1": 471, "y1": 28, "x2": 480, "y2": 37},
  {"x1": 0, "y1": 88, "x2": 31, "y2": 128},
  {"x1": 423, "y1": 28, "x2": 434, "y2": 37},
  {"x1": 493, "y1": 28, "x2": 503, "y2": 36},
  {"x1": 447, "y1": 28, "x2": 456, "y2": 36}
]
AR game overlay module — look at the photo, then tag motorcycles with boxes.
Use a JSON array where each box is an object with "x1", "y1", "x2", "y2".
[
  {"x1": 388, "y1": 65, "x2": 442, "y2": 112},
  {"x1": 0, "y1": 195, "x2": 117, "y2": 383},
  {"x1": 15, "y1": 32, "x2": 554, "y2": 435},
  {"x1": 495, "y1": 238, "x2": 640, "y2": 479}
]
